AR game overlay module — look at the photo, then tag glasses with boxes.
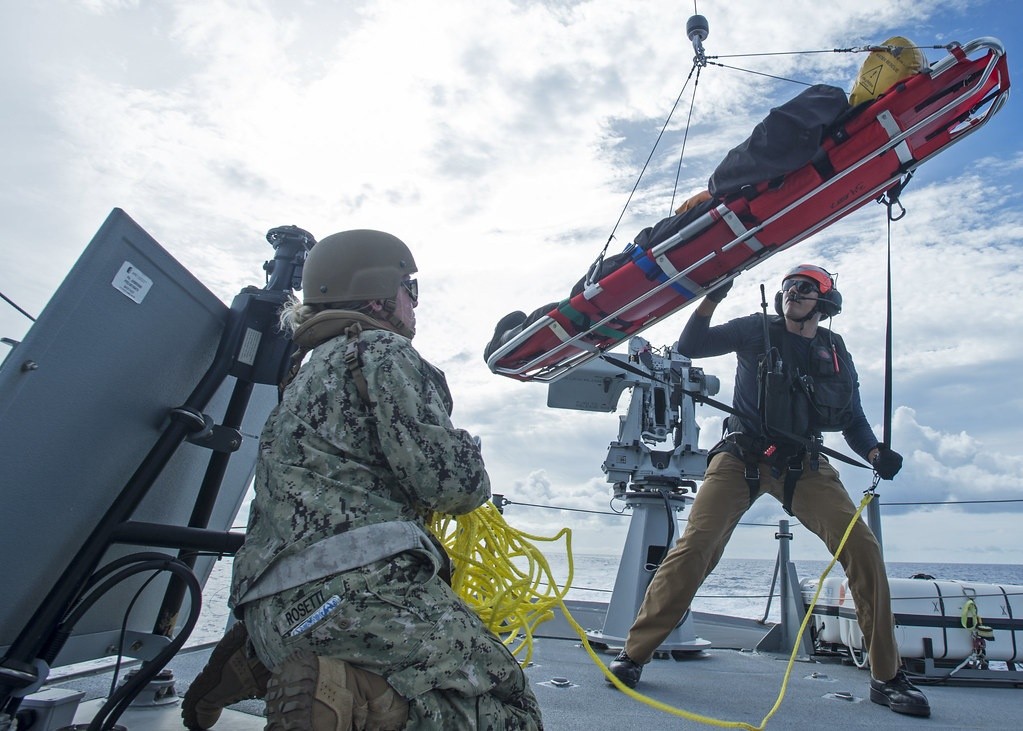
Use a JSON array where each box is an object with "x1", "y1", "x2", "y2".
[
  {"x1": 400, "y1": 279, "x2": 418, "y2": 301},
  {"x1": 782, "y1": 279, "x2": 820, "y2": 294}
]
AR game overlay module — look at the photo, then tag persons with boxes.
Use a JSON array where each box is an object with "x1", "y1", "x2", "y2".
[
  {"x1": 179, "y1": 229, "x2": 542, "y2": 731},
  {"x1": 602, "y1": 264, "x2": 935, "y2": 722}
]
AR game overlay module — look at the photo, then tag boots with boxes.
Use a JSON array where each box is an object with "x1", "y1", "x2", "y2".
[
  {"x1": 263, "y1": 648, "x2": 409, "y2": 731},
  {"x1": 484, "y1": 311, "x2": 527, "y2": 363},
  {"x1": 180, "y1": 620, "x2": 269, "y2": 731}
]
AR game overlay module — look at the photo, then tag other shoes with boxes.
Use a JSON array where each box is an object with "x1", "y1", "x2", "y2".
[
  {"x1": 605, "y1": 649, "x2": 643, "y2": 686},
  {"x1": 869, "y1": 671, "x2": 930, "y2": 716}
]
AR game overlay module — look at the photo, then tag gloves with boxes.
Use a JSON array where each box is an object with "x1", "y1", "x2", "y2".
[
  {"x1": 706, "y1": 280, "x2": 733, "y2": 302},
  {"x1": 872, "y1": 443, "x2": 903, "y2": 481}
]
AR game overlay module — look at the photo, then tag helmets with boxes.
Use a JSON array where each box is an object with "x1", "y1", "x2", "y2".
[
  {"x1": 781, "y1": 265, "x2": 833, "y2": 294},
  {"x1": 302, "y1": 229, "x2": 418, "y2": 305}
]
